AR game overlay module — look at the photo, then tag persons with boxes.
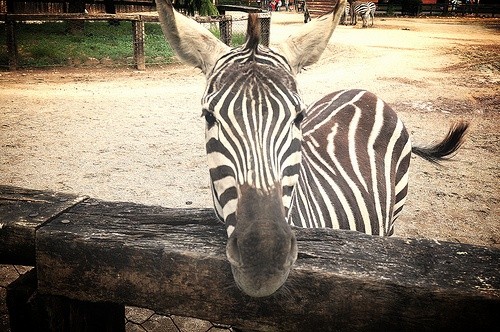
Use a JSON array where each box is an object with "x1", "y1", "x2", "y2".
[{"x1": 267, "y1": 0, "x2": 289, "y2": 11}]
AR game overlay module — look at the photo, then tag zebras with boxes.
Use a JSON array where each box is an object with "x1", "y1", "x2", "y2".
[
  {"x1": 340, "y1": 2, "x2": 376, "y2": 28},
  {"x1": 151, "y1": 0, "x2": 472, "y2": 299}
]
